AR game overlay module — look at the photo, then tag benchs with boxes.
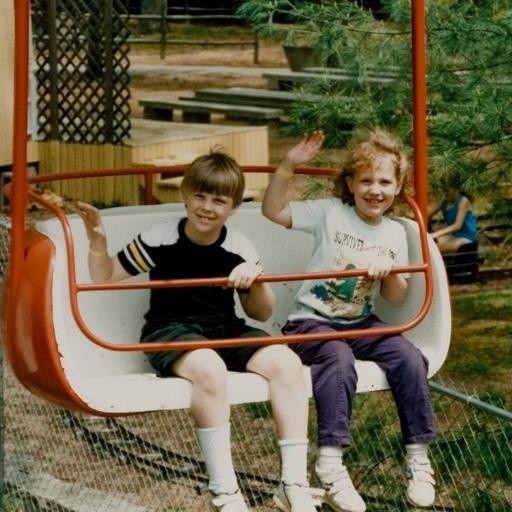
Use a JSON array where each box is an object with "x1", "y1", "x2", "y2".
[{"x1": 139, "y1": 64, "x2": 512, "y2": 126}]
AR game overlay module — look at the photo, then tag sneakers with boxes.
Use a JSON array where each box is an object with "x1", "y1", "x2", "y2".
[
  {"x1": 310, "y1": 462, "x2": 367, "y2": 512},
  {"x1": 208, "y1": 491, "x2": 251, "y2": 512},
  {"x1": 272, "y1": 484, "x2": 325, "y2": 512},
  {"x1": 404, "y1": 454, "x2": 437, "y2": 508}
]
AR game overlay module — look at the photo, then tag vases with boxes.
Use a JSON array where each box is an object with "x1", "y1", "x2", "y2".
[
  {"x1": 282, "y1": 44, "x2": 321, "y2": 72},
  {"x1": 328, "y1": 46, "x2": 355, "y2": 67}
]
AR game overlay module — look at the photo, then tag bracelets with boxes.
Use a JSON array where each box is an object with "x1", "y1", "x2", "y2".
[
  {"x1": 275, "y1": 167, "x2": 294, "y2": 179},
  {"x1": 88, "y1": 250, "x2": 107, "y2": 257}
]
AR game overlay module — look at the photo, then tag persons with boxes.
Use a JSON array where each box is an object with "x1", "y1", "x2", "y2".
[
  {"x1": 261, "y1": 129, "x2": 438, "y2": 512},
  {"x1": 426, "y1": 187, "x2": 479, "y2": 284},
  {"x1": 69, "y1": 150, "x2": 316, "y2": 512}
]
